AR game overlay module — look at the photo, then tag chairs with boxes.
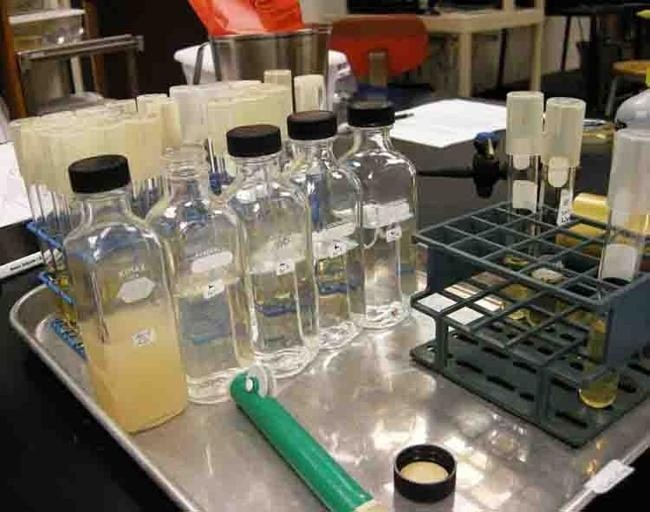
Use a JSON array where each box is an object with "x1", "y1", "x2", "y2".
[{"x1": 330, "y1": 13, "x2": 437, "y2": 111}]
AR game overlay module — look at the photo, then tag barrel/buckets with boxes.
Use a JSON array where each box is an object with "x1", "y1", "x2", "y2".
[{"x1": 209, "y1": 22, "x2": 332, "y2": 82}]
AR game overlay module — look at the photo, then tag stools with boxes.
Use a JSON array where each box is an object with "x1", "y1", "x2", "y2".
[{"x1": 605, "y1": 60, "x2": 650, "y2": 115}]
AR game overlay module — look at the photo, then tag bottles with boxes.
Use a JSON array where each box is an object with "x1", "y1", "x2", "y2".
[
  {"x1": 499, "y1": 89, "x2": 544, "y2": 319},
  {"x1": 220, "y1": 121, "x2": 323, "y2": 382},
  {"x1": 8, "y1": 68, "x2": 328, "y2": 328},
  {"x1": 61, "y1": 153, "x2": 189, "y2": 436},
  {"x1": 145, "y1": 142, "x2": 259, "y2": 406},
  {"x1": 578, "y1": 87, "x2": 650, "y2": 409},
  {"x1": 523, "y1": 96, "x2": 587, "y2": 330},
  {"x1": 282, "y1": 108, "x2": 369, "y2": 349},
  {"x1": 336, "y1": 97, "x2": 419, "y2": 332}
]
